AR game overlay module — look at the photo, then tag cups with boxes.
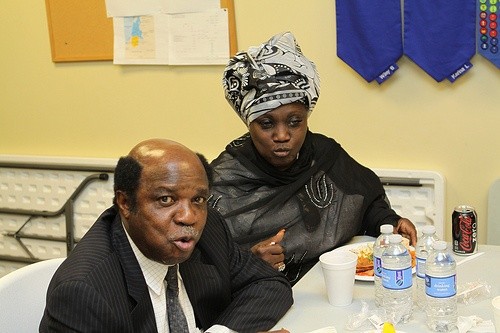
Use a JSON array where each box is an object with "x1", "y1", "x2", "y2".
[
  {"x1": 491, "y1": 296, "x2": 500, "y2": 333},
  {"x1": 319, "y1": 249, "x2": 358, "y2": 307}
]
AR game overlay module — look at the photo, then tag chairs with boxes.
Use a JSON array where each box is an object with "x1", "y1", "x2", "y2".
[{"x1": 0, "y1": 257, "x2": 67, "y2": 332}]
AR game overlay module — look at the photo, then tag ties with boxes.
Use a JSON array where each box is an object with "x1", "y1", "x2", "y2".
[{"x1": 165, "y1": 264, "x2": 189, "y2": 333}]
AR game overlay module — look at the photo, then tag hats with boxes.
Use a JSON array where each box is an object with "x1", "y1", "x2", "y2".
[{"x1": 222, "y1": 32, "x2": 321, "y2": 127}]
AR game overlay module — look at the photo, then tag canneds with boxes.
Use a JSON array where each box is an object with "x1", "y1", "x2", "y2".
[{"x1": 451, "y1": 205, "x2": 477, "y2": 256}]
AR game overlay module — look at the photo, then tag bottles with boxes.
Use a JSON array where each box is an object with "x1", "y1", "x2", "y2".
[
  {"x1": 372, "y1": 224, "x2": 394, "y2": 310},
  {"x1": 424, "y1": 240, "x2": 459, "y2": 333},
  {"x1": 415, "y1": 225, "x2": 441, "y2": 311},
  {"x1": 381, "y1": 234, "x2": 415, "y2": 326}
]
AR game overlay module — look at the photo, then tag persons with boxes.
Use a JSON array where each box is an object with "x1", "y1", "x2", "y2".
[
  {"x1": 39, "y1": 139, "x2": 296, "y2": 333},
  {"x1": 207, "y1": 32, "x2": 417, "y2": 291}
]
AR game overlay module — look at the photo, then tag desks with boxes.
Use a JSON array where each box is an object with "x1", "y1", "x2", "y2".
[{"x1": 267, "y1": 244, "x2": 500, "y2": 333}]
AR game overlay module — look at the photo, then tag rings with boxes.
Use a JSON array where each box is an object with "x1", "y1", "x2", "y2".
[{"x1": 277, "y1": 264, "x2": 285, "y2": 271}]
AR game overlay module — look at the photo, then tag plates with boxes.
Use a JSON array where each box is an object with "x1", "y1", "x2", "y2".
[{"x1": 333, "y1": 241, "x2": 416, "y2": 282}]
